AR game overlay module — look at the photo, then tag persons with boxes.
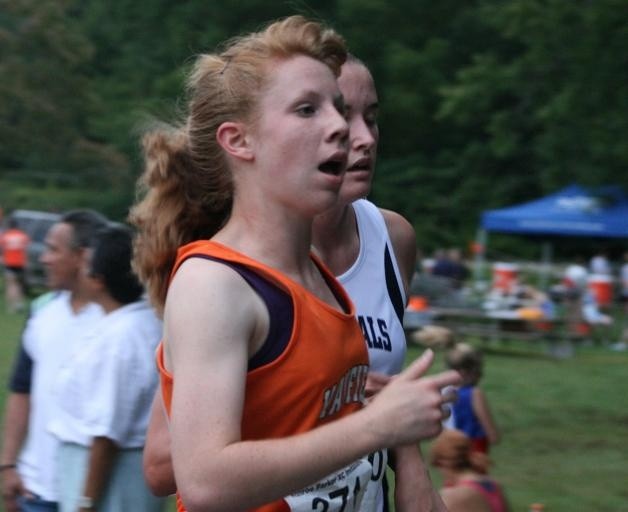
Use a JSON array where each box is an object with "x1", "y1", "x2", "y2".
[
  {"x1": 1, "y1": 210, "x2": 168, "y2": 512},
  {"x1": 430, "y1": 342, "x2": 507, "y2": 512},
  {"x1": 420, "y1": 247, "x2": 628, "y2": 329}
]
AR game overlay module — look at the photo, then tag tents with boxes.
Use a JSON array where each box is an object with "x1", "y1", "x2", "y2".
[{"x1": 475, "y1": 187, "x2": 628, "y2": 277}]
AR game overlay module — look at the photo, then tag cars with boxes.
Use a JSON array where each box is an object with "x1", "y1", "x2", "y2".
[{"x1": 0, "y1": 210, "x2": 62, "y2": 271}]
